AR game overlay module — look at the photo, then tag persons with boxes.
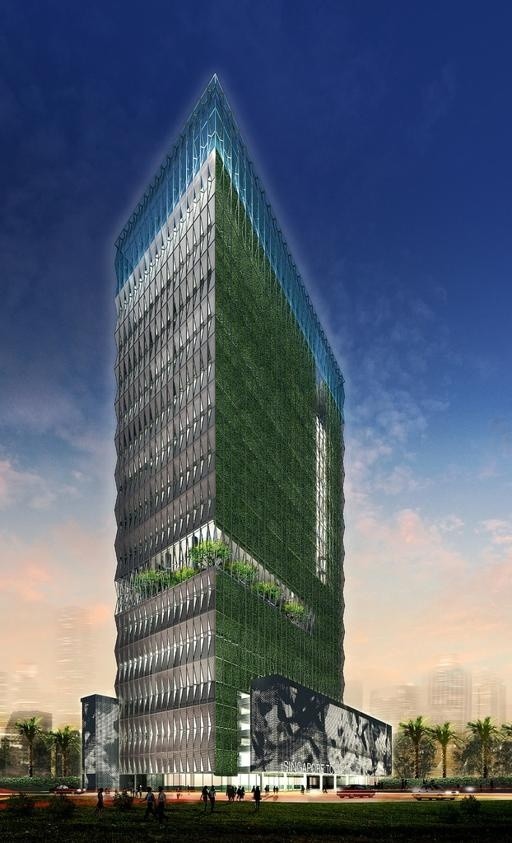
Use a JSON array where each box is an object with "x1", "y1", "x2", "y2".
[
  {"x1": 372, "y1": 778, "x2": 407, "y2": 791},
  {"x1": 91, "y1": 784, "x2": 307, "y2": 823}
]
